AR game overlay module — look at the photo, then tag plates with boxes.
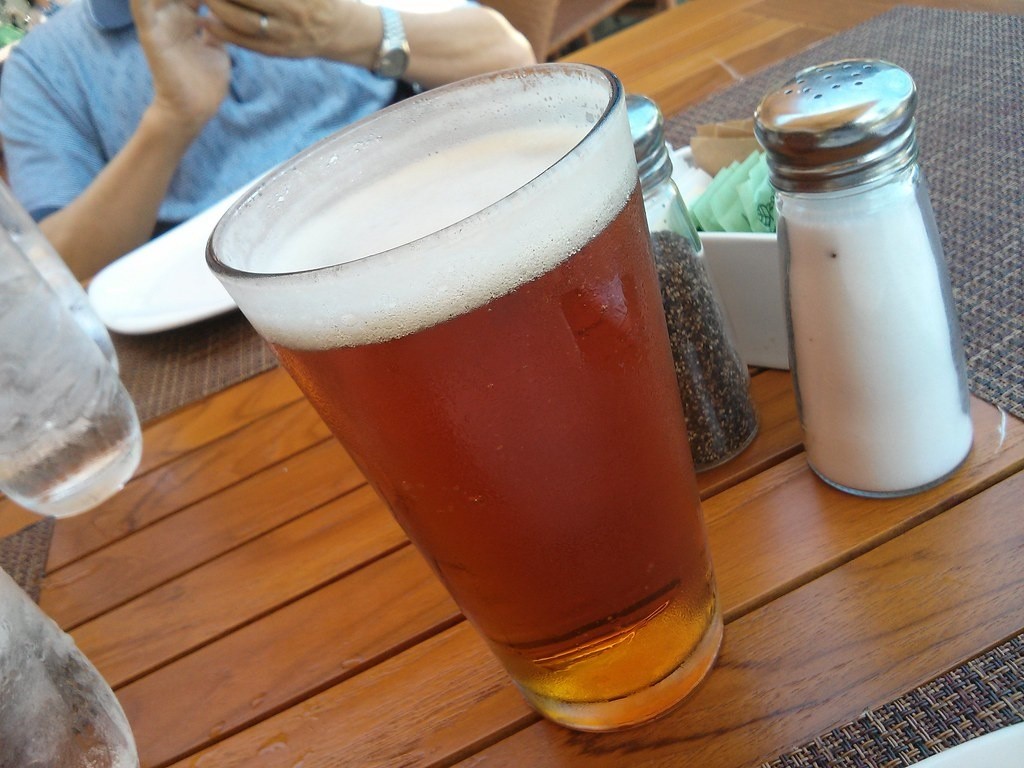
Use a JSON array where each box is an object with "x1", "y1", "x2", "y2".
[{"x1": 86, "y1": 160, "x2": 289, "y2": 334}]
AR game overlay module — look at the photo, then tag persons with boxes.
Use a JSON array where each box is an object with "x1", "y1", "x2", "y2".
[{"x1": 0, "y1": 0, "x2": 538, "y2": 278}]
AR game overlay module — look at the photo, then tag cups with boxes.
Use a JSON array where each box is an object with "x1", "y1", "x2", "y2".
[
  {"x1": 0, "y1": 568, "x2": 140, "y2": 768},
  {"x1": 203, "y1": 60, "x2": 724, "y2": 735},
  {"x1": 0, "y1": 187, "x2": 144, "y2": 523}
]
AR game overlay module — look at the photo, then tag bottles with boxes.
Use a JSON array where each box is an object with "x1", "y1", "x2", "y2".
[
  {"x1": 624, "y1": 92, "x2": 760, "y2": 473},
  {"x1": 753, "y1": 58, "x2": 974, "y2": 497}
]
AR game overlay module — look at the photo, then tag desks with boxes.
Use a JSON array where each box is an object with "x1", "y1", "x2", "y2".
[{"x1": 0, "y1": 0, "x2": 1024, "y2": 768}]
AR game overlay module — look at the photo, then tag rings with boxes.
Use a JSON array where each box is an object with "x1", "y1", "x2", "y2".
[{"x1": 259, "y1": 16, "x2": 268, "y2": 37}]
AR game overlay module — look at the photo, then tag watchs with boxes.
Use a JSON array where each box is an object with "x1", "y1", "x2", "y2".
[{"x1": 370, "y1": 6, "x2": 410, "y2": 79}]
